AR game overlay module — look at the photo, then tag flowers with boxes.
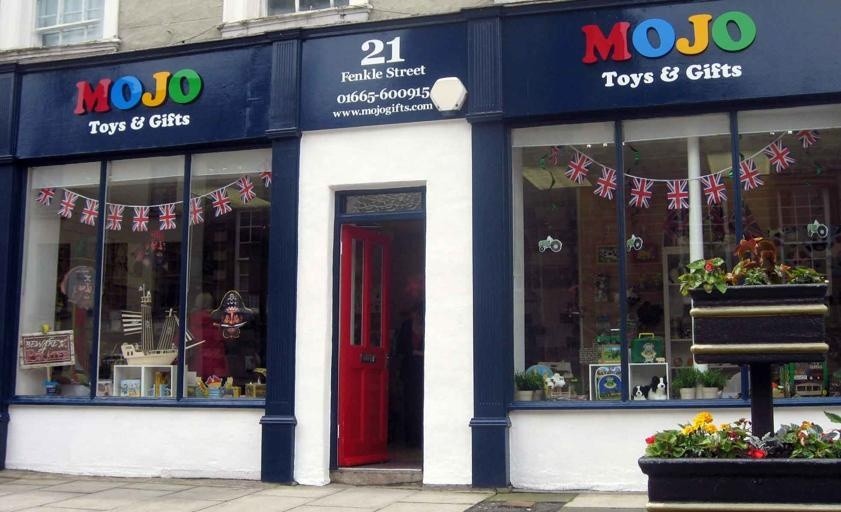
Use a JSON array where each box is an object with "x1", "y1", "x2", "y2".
[{"x1": 645, "y1": 412, "x2": 841, "y2": 458}]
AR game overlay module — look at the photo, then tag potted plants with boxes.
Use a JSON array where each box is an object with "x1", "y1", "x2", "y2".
[
  {"x1": 515, "y1": 370, "x2": 535, "y2": 401},
  {"x1": 672, "y1": 368, "x2": 726, "y2": 400},
  {"x1": 678, "y1": 237, "x2": 830, "y2": 363},
  {"x1": 535, "y1": 372, "x2": 544, "y2": 400}
]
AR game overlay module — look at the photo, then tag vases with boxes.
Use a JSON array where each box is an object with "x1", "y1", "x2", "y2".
[{"x1": 639, "y1": 456, "x2": 841, "y2": 512}]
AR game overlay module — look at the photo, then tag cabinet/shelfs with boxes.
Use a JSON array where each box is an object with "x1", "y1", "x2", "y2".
[{"x1": 662, "y1": 247, "x2": 738, "y2": 398}]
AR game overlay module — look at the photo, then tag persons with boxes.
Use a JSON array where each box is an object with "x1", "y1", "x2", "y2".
[
  {"x1": 61, "y1": 266, "x2": 96, "y2": 310},
  {"x1": 189, "y1": 293, "x2": 229, "y2": 379},
  {"x1": 401, "y1": 300, "x2": 423, "y2": 449},
  {"x1": 210, "y1": 290, "x2": 258, "y2": 340}
]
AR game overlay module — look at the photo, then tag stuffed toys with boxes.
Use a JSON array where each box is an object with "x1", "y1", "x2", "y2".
[
  {"x1": 646, "y1": 376, "x2": 667, "y2": 400},
  {"x1": 633, "y1": 385, "x2": 647, "y2": 400}
]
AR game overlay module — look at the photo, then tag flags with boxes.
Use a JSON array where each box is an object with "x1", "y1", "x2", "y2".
[
  {"x1": 34, "y1": 172, "x2": 272, "y2": 232},
  {"x1": 545, "y1": 130, "x2": 821, "y2": 210}
]
